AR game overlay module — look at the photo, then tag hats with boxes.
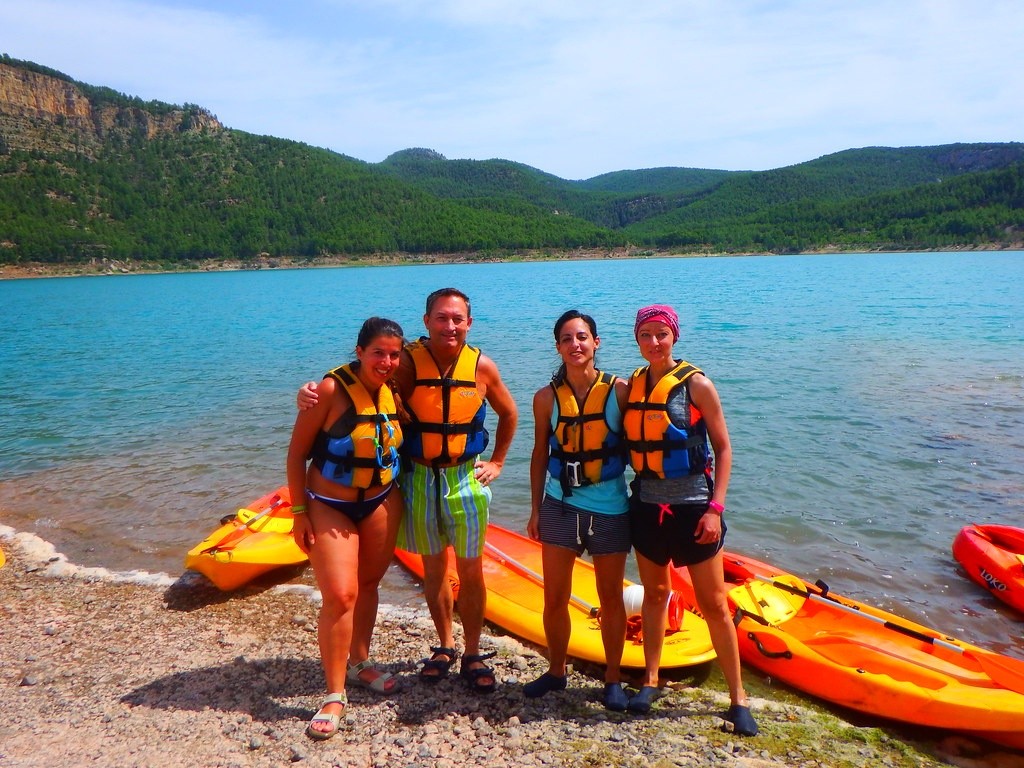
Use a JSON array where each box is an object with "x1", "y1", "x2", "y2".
[{"x1": 635, "y1": 305, "x2": 678, "y2": 344}]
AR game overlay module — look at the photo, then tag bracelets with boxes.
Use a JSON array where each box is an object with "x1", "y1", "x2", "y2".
[
  {"x1": 709, "y1": 500, "x2": 724, "y2": 513},
  {"x1": 288, "y1": 505, "x2": 308, "y2": 515}
]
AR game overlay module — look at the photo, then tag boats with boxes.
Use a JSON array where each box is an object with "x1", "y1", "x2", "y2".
[
  {"x1": 185, "y1": 484, "x2": 310, "y2": 592},
  {"x1": 671, "y1": 548, "x2": 1024, "y2": 751},
  {"x1": 951, "y1": 522, "x2": 1024, "y2": 615}
]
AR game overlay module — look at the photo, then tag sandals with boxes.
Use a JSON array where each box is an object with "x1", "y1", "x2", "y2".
[
  {"x1": 459, "y1": 650, "x2": 498, "y2": 694},
  {"x1": 345, "y1": 659, "x2": 403, "y2": 697},
  {"x1": 417, "y1": 647, "x2": 459, "y2": 684},
  {"x1": 308, "y1": 689, "x2": 348, "y2": 739}
]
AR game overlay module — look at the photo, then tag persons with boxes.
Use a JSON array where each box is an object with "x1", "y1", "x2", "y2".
[
  {"x1": 296, "y1": 287, "x2": 518, "y2": 695},
  {"x1": 522, "y1": 311, "x2": 631, "y2": 712},
  {"x1": 624, "y1": 304, "x2": 759, "y2": 738},
  {"x1": 286, "y1": 316, "x2": 410, "y2": 739}
]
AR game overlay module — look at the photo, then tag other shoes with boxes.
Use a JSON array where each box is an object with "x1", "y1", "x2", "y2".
[
  {"x1": 629, "y1": 685, "x2": 661, "y2": 713},
  {"x1": 603, "y1": 683, "x2": 630, "y2": 711},
  {"x1": 524, "y1": 670, "x2": 568, "y2": 695},
  {"x1": 728, "y1": 705, "x2": 758, "y2": 737}
]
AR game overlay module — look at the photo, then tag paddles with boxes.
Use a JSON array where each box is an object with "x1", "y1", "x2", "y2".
[
  {"x1": 723, "y1": 558, "x2": 1024, "y2": 694},
  {"x1": 238, "y1": 484, "x2": 293, "y2": 531},
  {"x1": 481, "y1": 541, "x2": 601, "y2": 619}
]
